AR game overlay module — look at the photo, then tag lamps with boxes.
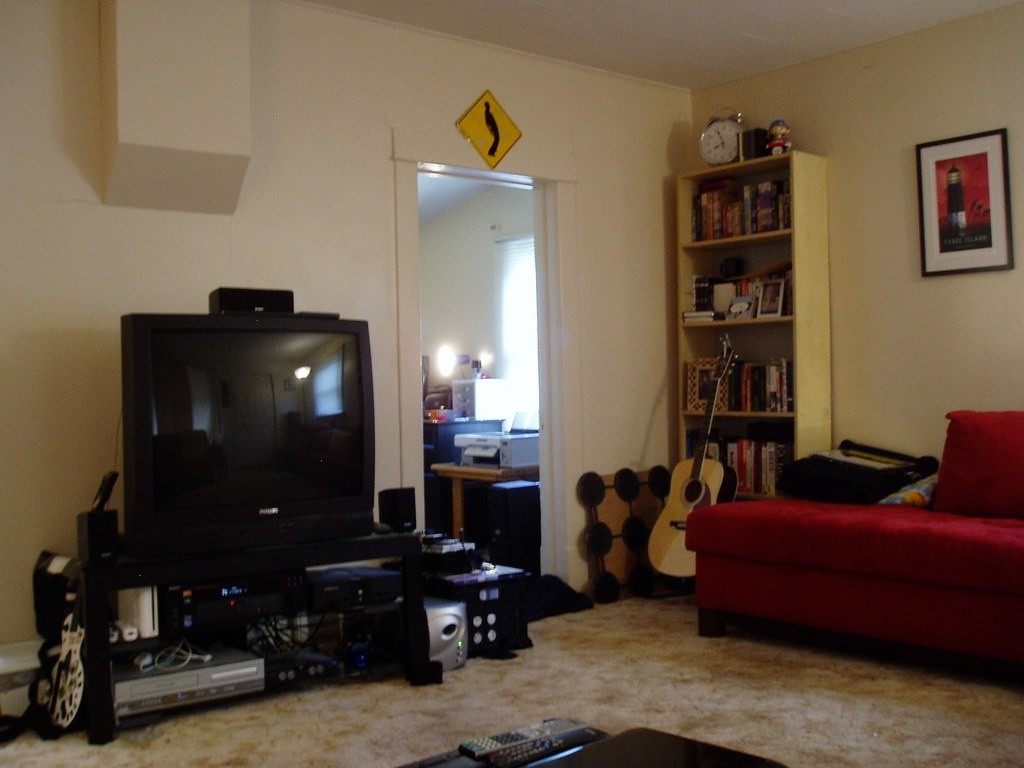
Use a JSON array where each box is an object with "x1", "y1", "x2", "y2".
[{"x1": 437, "y1": 345, "x2": 470, "y2": 380}]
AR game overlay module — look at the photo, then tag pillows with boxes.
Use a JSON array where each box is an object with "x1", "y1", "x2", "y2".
[{"x1": 932, "y1": 410, "x2": 1024, "y2": 516}]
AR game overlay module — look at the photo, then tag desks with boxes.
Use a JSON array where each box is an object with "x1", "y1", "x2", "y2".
[{"x1": 430, "y1": 463, "x2": 539, "y2": 544}]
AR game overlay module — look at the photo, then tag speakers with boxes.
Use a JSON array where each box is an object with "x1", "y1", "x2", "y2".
[
  {"x1": 208, "y1": 287, "x2": 295, "y2": 314},
  {"x1": 77, "y1": 508, "x2": 119, "y2": 563},
  {"x1": 377, "y1": 487, "x2": 417, "y2": 534}
]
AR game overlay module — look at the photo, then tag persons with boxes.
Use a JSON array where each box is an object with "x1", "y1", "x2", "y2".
[
  {"x1": 698, "y1": 371, "x2": 711, "y2": 399},
  {"x1": 763, "y1": 285, "x2": 779, "y2": 313}
]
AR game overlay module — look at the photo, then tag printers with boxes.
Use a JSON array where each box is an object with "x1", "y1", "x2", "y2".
[{"x1": 454, "y1": 432, "x2": 539, "y2": 469}]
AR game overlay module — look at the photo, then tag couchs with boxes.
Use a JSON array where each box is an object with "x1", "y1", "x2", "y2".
[{"x1": 685, "y1": 498, "x2": 1024, "y2": 662}]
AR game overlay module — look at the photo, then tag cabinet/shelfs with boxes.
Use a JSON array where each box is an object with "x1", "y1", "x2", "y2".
[
  {"x1": 73, "y1": 533, "x2": 430, "y2": 746},
  {"x1": 676, "y1": 150, "x2": 832, "y2": 502}
]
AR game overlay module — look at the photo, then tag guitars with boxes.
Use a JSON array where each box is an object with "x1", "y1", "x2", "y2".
[
  {"x1": 49, "y1": 470, "x2": 125, "y2": 735},
  {"x1": 646, "y1": 334, "x2": 748, "y2": 580}
]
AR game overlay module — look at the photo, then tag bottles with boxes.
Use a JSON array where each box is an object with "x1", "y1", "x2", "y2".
[{"x1": 946, "y1": 164, "x2": 967, "y2": 238}]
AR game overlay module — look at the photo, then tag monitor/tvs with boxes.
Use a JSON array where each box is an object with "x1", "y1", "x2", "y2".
[{"x1": 121, "y1": 311, "x2": 375, "y2": 553}]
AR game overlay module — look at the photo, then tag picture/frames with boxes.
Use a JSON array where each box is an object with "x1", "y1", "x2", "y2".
[
  {"x1": 915, "y1": 128, "x2": 1014, "y2": 276},
  {"x1": 687, "y1": 358, "x2": 728, "y2": 413},
  {"x1": 756, "y1": 279, "x2": 785, "y2": 319}
]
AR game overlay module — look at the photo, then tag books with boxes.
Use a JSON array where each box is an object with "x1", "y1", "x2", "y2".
[
  {"x1": 685, "y1": 427, "x2": 794, "y2": 500},
  {"x1": 724, "y1": 354, "x2": 794, "y2": 412},
  {"x1": 682, "y1": 174, "x2": 790, "y2": 245},
  {"x1": 678, "y1": 259, "x2": 791, "y2": 327}
]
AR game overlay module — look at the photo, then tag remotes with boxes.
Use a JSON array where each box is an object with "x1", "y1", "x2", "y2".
[{"x1": 458, "y1": 719, "x2": 607, "y2": 768}]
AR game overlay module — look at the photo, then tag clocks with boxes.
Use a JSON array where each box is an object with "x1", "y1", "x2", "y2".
[{"x1": 699, "y1": 107, "x2": 744, "y2": 167}]
